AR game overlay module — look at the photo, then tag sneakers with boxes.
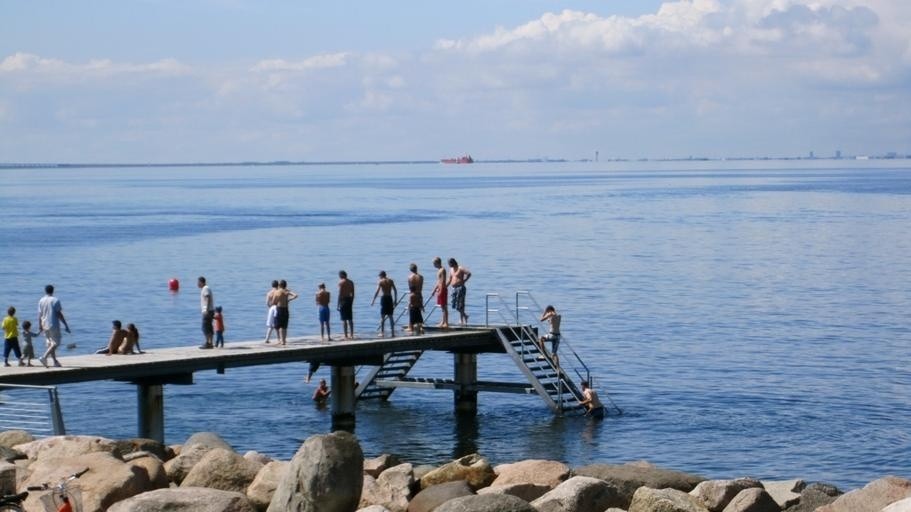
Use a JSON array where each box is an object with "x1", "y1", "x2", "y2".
[
  {"x1": 199, "y1": 344, "x2": 213, "y2": 349},
  {"x1": 4, "y1": 362, "x2": 33, "y2": 367},
  {"x1": 54, "y1": 362, "x2": 61, "y2": 367},
  {"x1": 39, "y1": 357, "x2": 48, "y2": 368}
]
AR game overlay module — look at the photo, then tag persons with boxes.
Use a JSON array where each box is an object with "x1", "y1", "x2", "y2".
[
  {"x1": 271, "y1": 279, "x2": 299, "y2": 345},
  {"x1": 198, "y1": 276, "x2": 213, "y2": 349},
  {"x1": 117, "y1": 323, "x2": 147, "y2": 354},
  {"x1": 17, "y1": 320, "x2": 42, "y2": 367},
  {"x1": 408, "y1": 285, "x2": 425, "y2": 335},
  {"x1": 578, "y1": 380, "x2": 604, "y2": 420},
  {"x1": 371, "y1": 270, "x2": 400, "y2": 338},
  {"x1": 313, "y1": 378, "x2": 332, "y2": 402},
  {"x1": 446, "y1": 258, "x2": 472, "y2": 326},
  {"x1": 432, "y1": 256, "x2": 449, "y2": 330},
  {"x1": 403, "y1": 262, "x2": 424, "y2": 332},
  {"x1": 315, "y1": 282, "x2": 333, "y2": 341},
  {"x1": 337, "y1": 269, "x2": 356, "y2": 341},
  {"x1": 303, "y1": 361, "x2": 320, "y2": 384},
  {"x1": 2, "y1": 306, "x2": 26, "y2": 367},
  {"x1": 38, "y1": 284, "x2": 72, "y2": 369},
  {"x1": 539, "y1": 305, "x2": 562, "y2": 370},
  {"x1": 209, "y1": 304, "x2": 225, "y2": 348},
  {"x1": 265, "y1": 280, "x2": 279, "y2": 344},
  {"x1": 95, "y1": 320, "x2": 124, "y2": 356}
]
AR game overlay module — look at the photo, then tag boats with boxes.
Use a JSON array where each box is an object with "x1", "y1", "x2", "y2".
[{"x1": 441, "y1": 151, "x2": 474, "y2": 163}]
